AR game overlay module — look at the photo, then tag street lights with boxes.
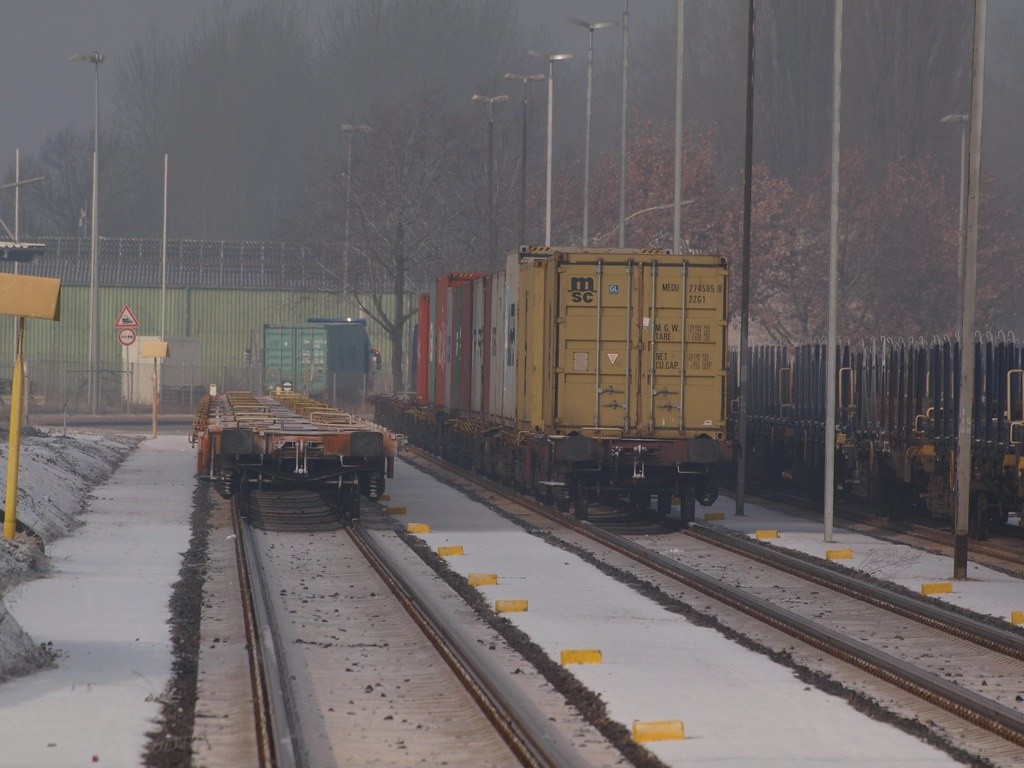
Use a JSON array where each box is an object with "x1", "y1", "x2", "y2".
[
  {"x1": 68, "y1": 49, "x2": 110, "y2": 401},
  {"x1": 461, "y1": 0, "x2": 612, "y2": 247}
]
